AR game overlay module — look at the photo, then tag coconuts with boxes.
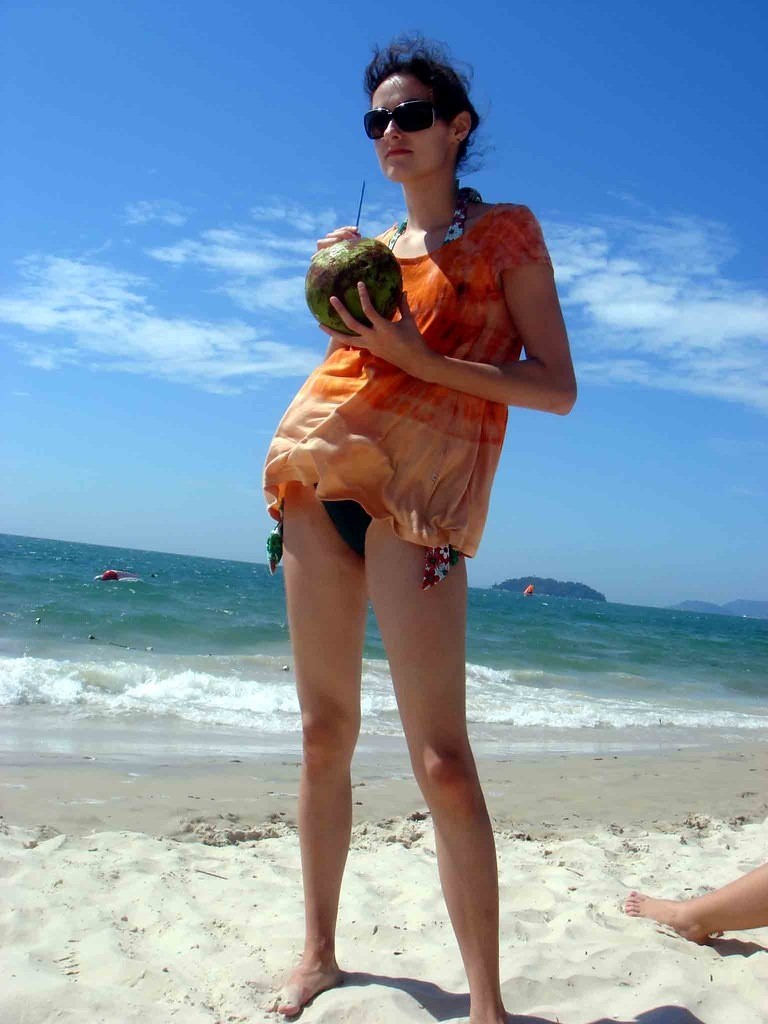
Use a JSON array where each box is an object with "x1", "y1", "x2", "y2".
[{"x1": 304, "y1": 237, "x2": 403, "y2": 334}]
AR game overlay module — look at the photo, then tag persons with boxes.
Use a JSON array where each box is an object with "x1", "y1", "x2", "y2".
[
  {"x1": 624, "y1": 859, "x2": 768, "y2": 947},
  {"x1": 262, "y1": 37, "x2": 577, "y2": 1023}
]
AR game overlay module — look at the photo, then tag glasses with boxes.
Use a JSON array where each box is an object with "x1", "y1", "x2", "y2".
[{"x1": 364, "y1": 100, "x2": 438, "y2": 139}]
